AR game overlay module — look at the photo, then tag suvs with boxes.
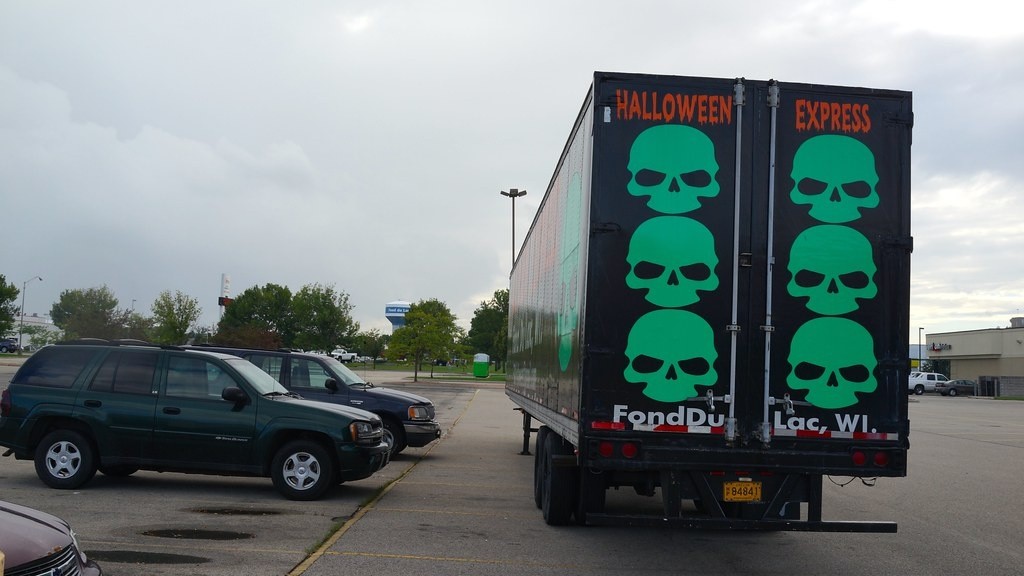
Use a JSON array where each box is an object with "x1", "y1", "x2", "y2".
[{"x1": 0, "y1": 337, "x2": 442, "y2": 500}]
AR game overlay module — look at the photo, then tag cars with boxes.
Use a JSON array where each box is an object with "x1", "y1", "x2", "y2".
[
  {"x1": 0, "y1": 502, "x2": 103, "y2": 576},
  {"x1": 0, "y1": 338, "x2": 19, "y2": 353},
  {"x1": 360, "y1": 355, "x2": 389, "y2": 364},
  {"x1": 908, "y1": 372, "x2": 949, "y2": 395},
  {"x1": 940, "y1": 378, "x2": 984, "y2": 396}
]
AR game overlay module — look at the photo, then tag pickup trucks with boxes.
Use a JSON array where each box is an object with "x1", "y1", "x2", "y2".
[{"x1": 330, "y1": 348, "x2": 358, "y2": 363}]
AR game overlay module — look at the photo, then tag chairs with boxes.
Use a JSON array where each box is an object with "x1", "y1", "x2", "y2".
[
  {"x1": 181, "y1": 368, "x2": 210, "y2": 400},
  {"x1": 290, "y1": 366, "x2": 310, "y2": 387}
]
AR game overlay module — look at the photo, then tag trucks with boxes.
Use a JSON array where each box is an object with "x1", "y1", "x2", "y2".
[{"x1": 503, "y1": 70, "x2": 915, "y2": 533}]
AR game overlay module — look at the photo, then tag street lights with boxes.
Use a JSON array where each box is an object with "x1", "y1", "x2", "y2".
[
  {"x1": 19, "y1": 275, "x2": 42, "y2": 356},
  {"x1": 917, "y1": 327, "x2": 924, "y2": 371},
  {"x1": 499, "y1": 189, "x2": 527, "y2": 267},
  {"x1": 130, "y1": 300, "x2": 137, "y2": 326}
]
